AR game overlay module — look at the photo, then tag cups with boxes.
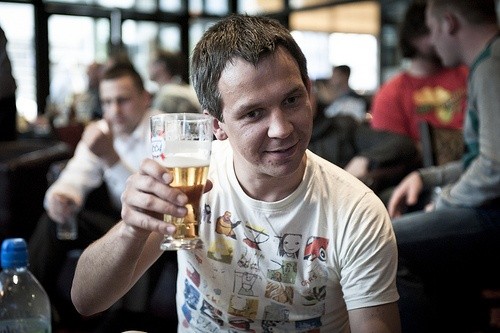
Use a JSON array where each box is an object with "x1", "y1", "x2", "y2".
[
  {"x1": 57, "y1": 205, "x2": 77, "y2": 240},
  {"x1": 150, "y1": 112, "x2": 213, "y2": 250}
]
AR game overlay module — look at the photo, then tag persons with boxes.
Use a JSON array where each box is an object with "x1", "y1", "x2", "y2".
[
  {"x1": 70, "y1": 13, "x2": 401, "y2": 333},
  {"x1": 1, "y1": 0, "x2": 500, "y2": 333}
]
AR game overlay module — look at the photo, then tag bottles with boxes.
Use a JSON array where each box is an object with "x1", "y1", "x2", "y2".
[{"x1": 0, "y1": 238, "x2": 51, "y2": 333}]
